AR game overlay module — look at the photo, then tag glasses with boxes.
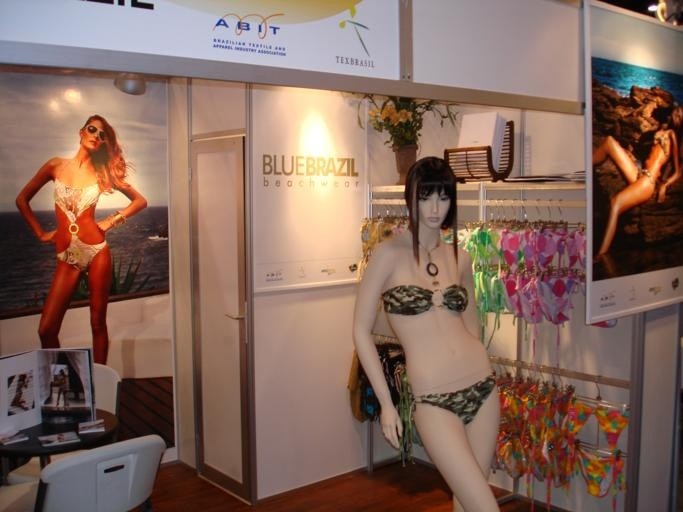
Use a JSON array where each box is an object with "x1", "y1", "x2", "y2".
[{"x1": 83, "y1": 123, "x2": 108, "y2": 144}]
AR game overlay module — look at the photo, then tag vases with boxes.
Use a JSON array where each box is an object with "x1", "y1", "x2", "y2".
[{"x1": 389, "y1": 135, "x2": 420, "y2": 184}]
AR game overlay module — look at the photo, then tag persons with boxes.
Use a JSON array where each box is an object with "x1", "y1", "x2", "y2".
[
  {"x1": 352, "y1": 157, "x2": 502, "y2": 512},
  {"x1": 15, "y1": 114, "x2": 148, "y2": 366},
  {"x1": 54, "y1": 369, "x2": 69, "y2": 407},
  {"x1": 593, "y1": 108, "x2": 682, "y2": 255}
]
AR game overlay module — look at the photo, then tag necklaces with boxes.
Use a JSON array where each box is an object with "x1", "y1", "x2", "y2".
[{"x1": 418, "y1": 240, "x2": 440, "y2": 277}]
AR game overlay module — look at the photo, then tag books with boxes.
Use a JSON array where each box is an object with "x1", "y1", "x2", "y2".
[{"x1": 0, "y1": 348, "x2": 96, "y2": 442}]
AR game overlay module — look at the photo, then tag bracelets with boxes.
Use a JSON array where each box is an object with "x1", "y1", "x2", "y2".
[
  {"x1": 106, "y1": 216, "x2": 114, "y2": 227},
  {"x1": 113, "y1": 211, "x2": 126, "y2": 225}
]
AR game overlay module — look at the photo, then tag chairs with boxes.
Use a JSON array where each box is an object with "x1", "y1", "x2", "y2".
[
  {"x1": 31, "y1": 434, "x2": 165, "y2": 512},
  {"x1": 8, "y1": 362, "x2": 122, "y2": 483}
]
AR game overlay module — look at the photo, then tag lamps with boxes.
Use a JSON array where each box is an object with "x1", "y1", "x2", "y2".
[{"x1": 111, "y1": 72, "x2": 148, "y2": 96}]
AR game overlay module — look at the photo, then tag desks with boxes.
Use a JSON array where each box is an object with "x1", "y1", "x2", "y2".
[{"x1": 0, "y1": 409, "x2": 120, "y2": 472}]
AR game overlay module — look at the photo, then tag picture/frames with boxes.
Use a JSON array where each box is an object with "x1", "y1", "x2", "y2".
[{"x1": 579, "y1": 0, "x2": 682, "y2": 327}]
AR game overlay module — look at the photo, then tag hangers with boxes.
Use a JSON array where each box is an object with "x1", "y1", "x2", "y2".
[
  {"x1": 367, "y1": 333, "x2": 638, "y2": 463},
  {"x1": 372, "y1": 196, "x2": 586, "y2": 283}
]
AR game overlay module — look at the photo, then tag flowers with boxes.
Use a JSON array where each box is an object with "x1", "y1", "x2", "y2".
[{"x1": 358, "y1": 91, "x2": 456, "y2": 143}]
